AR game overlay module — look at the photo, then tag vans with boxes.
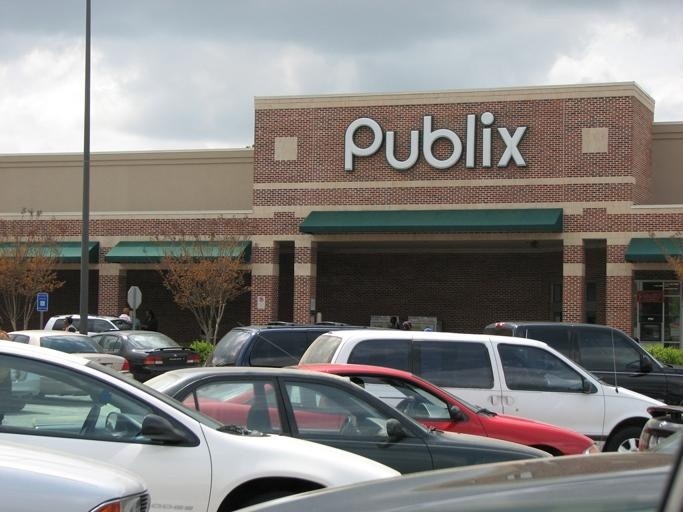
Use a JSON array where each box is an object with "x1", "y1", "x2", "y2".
[
  {"x1": 45, "y1": 313, "x2": 134, "y2": 336},
  {"x1": 288, "y1": 328, "x2": 669, "y2": 458}
]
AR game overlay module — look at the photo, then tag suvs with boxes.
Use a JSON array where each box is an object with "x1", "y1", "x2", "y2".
[
  {"x1": 208, "y1": 318, "x2": 366, "y2": 369},
  {"x1": 482, "y1": 322, "x2": 683, "y2": 405}
]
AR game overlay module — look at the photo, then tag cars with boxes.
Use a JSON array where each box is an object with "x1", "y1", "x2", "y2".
[
  {"x1": 136, "y1": 365, "x2": 552, "y2": 475},
  {"x1": 183, "y1": 362, "x2": 601, "y2": 455},
  {"x1": 1, "y1": 440, "x2": 153, "y2": 512},
  {"x1": 630, "y1": 402, "x2": 683, "y2": 451},
  {"x1": 92, "y1": 329, "x2": 201, "y2": 381},
  {"x1": 1, "y1": 340, "x2": 401, "y2": 512},
  {"x1": 227, "y1": 426, "x2": 683, "y2": 511},
  {"x1": 5, "y1": 327, "x2": 134, "y2": 415}
]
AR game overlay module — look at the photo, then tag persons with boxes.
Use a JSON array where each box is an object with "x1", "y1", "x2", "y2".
[
  {"x1": 64, "y1": 317, "x2": 77, "y2": 332},
  {"x1": 128, "y1": 310, "x2": 140, "y2": 330},
  {"x1": 141, "y1": 308, "x2": 157, "y2": 331},
  {"x1": 118, "y1": 307, "x2": 130, "y2": 322}
]
